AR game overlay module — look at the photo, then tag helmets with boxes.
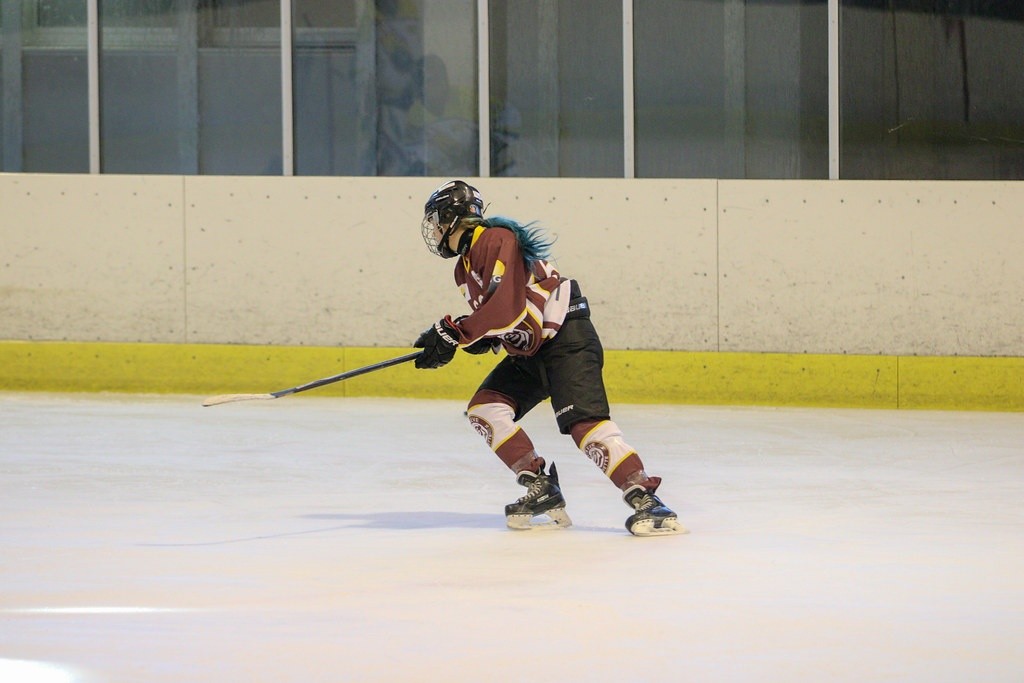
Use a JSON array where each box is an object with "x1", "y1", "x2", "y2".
[{"x1": 420, "y1": 180, "x2": 483, "y2": 259}]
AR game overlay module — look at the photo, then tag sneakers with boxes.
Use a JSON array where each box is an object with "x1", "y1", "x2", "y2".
[
  {"x1": 505, "y1": 461, "x2": 573, "y2": 529},
  {"x1": 622, "y1": 476, "x2": 686, "y2": 537}
]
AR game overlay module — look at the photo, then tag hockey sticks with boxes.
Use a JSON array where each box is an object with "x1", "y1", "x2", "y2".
[{"x1": 199, "y1": 335, "x2": 502, "y2": 410}]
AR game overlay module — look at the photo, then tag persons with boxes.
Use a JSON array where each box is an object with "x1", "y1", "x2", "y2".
[{"x1": 415, "y1": 180, "x2": 685, "y2": 537}]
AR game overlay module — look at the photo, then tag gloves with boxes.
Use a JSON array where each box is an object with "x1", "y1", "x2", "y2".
[
  {"x1": 413, "y1": 315, "x2": 460, "y2": 369},
  {"x1": 453, "y1": 314, "x2": 501, "y2": 355}
]
